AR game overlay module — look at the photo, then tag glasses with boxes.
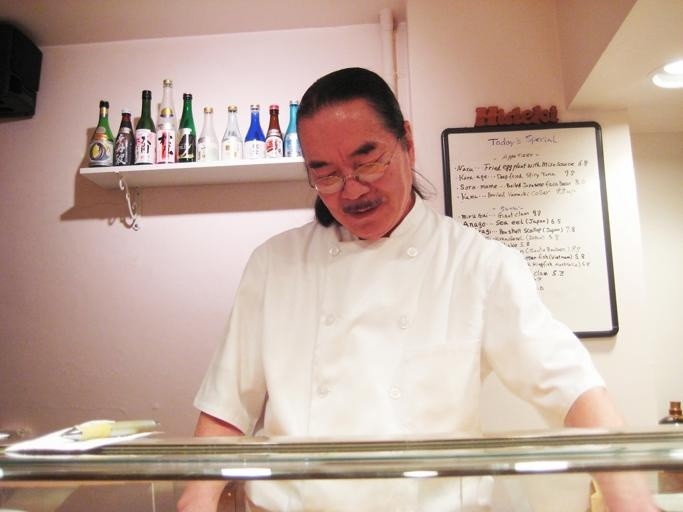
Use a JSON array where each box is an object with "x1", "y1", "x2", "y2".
[{"x1": 309, "y1": 139, "x2": 397, "y2": 194}]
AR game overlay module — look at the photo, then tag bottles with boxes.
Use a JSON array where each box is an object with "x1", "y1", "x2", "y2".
[
  {"x1": 87, "y1": 75, "x2": 302, "y2": 166},
  {"x1": 657, "y1": 399, "x2": 683, "y2": 495}
]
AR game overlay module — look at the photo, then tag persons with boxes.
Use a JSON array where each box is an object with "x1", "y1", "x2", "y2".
[{"x1": 174, "y1": 65, "x2": 661, "y2": 512}]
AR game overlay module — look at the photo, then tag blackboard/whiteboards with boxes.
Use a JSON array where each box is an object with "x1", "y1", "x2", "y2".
[{"x1": 440, "y1": 121, "x2": 619, "y2": 338}]
